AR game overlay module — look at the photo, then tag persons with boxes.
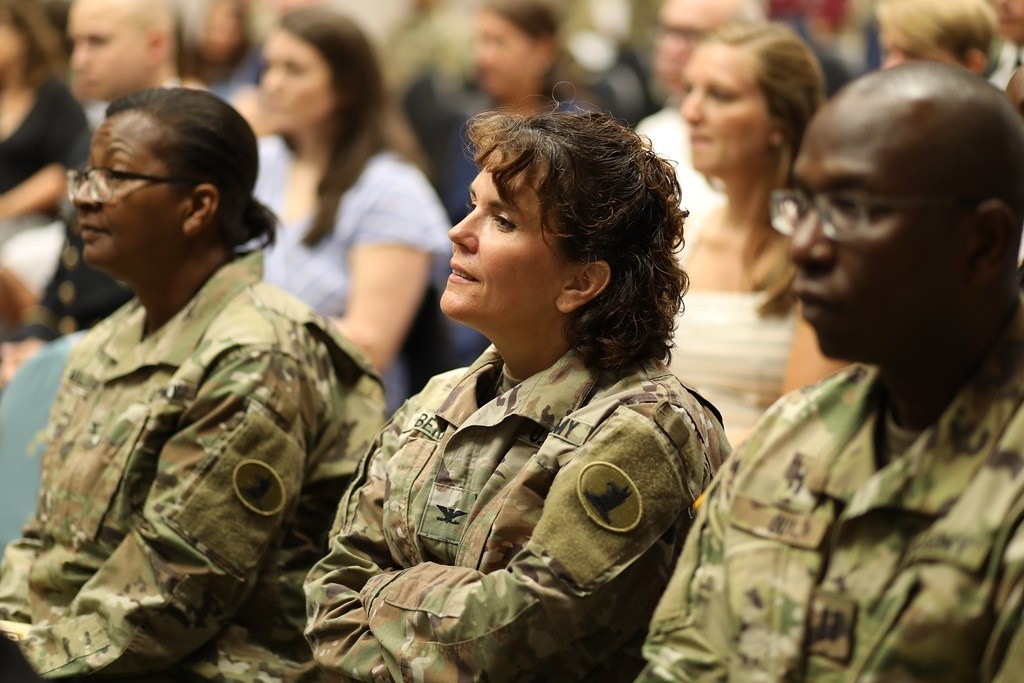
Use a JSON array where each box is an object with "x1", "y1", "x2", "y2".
[
  {"x1": 0, "y1": 0, "x2": 1024, "y2": 366},
  {"x1": 635, "y1": 63, "x2": 1024, "y2": 683},
  {"x1": 662, "y1": 21, "x2": 853, "y2": 446},
  {"x1": 247, "y1": 8, "x2": 454, "y2": 416},
  {"x1": 306, "y1": 105, "x2": 731, "y2": 683},
  {"x1": 0, "y1": 85, "x2": 385, "y2": 683}
]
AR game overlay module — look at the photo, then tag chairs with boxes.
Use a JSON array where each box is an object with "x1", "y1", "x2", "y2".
[{"x1": 0, "y1": 328, "x2": 91, "y2": 557}]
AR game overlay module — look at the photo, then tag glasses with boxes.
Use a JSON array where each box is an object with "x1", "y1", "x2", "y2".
[
  {"x1": 769, "y1": 188, "x2": 975, "y2": 242},
  {"x1": 65, "y1": 168, "x2": 195, "y2": 203}
]
